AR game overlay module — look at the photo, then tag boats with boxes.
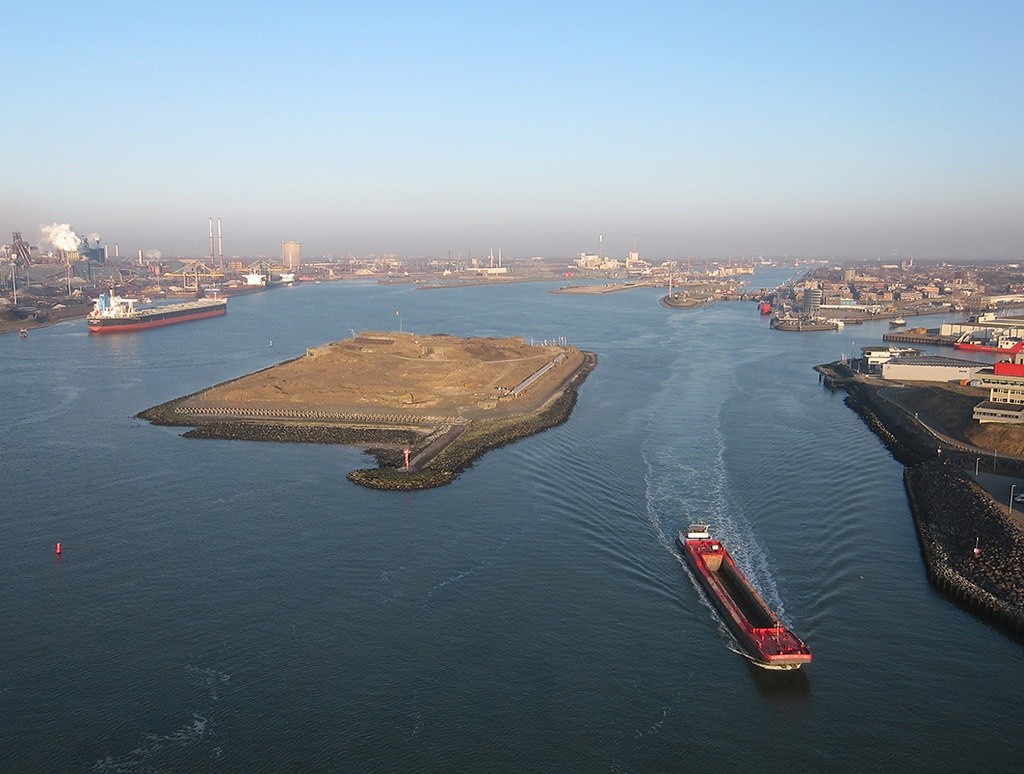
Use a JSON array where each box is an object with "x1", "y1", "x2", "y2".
[
  {"x1": 677, "y1": 522, "x2": 812, "y2": 670},
  {"x1": 84, "y1": 288, "x2": 226, "y2": 333}
]
